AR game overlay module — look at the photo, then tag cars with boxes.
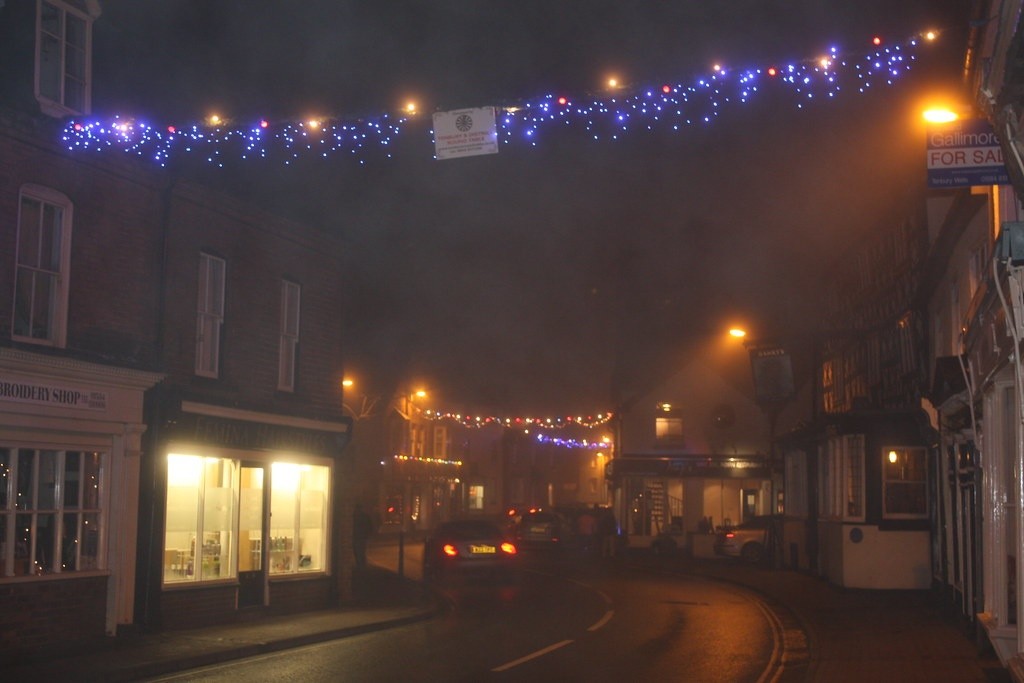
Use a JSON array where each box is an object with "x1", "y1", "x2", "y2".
[
  {"x1": 713, "y1": 511, "x2": 789, "y2": 558},
  {"x1": 521, "y1": 509, "x2": 569, "y2": 550},
  {"x1": 424, "y1": 518, "x2": 519, "y2": 583}
]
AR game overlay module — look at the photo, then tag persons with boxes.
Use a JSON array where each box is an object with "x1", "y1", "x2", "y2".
[
  {"x1": 574, "y1": 506, "x2": 619, "y2": 560},
  {"x1": 638, "y1": 489, "x2": 656, "y2": 537}
]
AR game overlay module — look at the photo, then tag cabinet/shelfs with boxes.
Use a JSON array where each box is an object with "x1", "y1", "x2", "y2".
[{"x1": 250, "y1": 538, "x2": 293, "y2": 570}]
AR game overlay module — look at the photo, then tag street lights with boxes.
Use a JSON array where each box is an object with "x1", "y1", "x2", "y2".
[
  {"x1": 729, "y1": 322, "x2": 788, "y2": 563},
  {"x1": 397, "y1": 386, "x2": 431, "y2": 584}
]
